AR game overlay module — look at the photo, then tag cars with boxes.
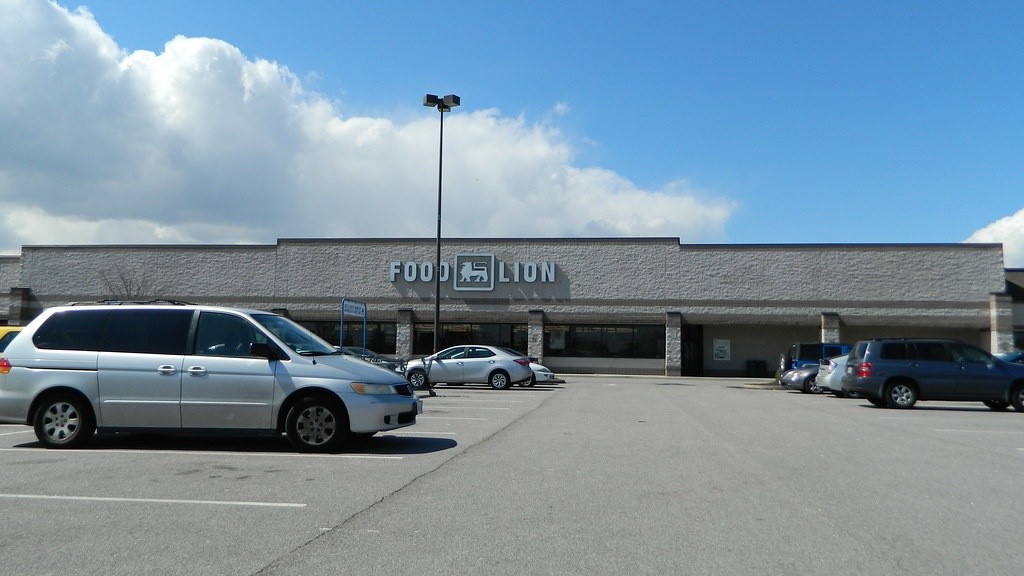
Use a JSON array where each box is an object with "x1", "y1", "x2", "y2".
[
  {"x1": 816, "y1": 355, "x2": 860, "y2": 398},
  {"x1": 779, "y1": 368, "x2": 824, "y2": 394},
  {"x1": 516, "y1": 361, "x2": 555, "y2": 386},
  {"x1": 404, "y1": 343, "x2": 533, "y2": 390}
]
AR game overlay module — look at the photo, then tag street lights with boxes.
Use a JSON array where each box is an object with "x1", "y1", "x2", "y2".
[{"x1": 423, "y1": 94, "x2": 461, "y2": 357}]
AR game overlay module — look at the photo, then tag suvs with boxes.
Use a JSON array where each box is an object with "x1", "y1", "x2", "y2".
[
  {"x1": 842, "y1": 337, "x2": 1024, "y2": 412},
  {"x1": 787, "y1": 343, "x2": 856, "y2": 373}
]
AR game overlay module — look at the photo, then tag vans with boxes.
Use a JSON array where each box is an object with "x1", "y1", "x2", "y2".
[{"x1": 0, "y1": 297, "x2": 424, "y2": 449}]
